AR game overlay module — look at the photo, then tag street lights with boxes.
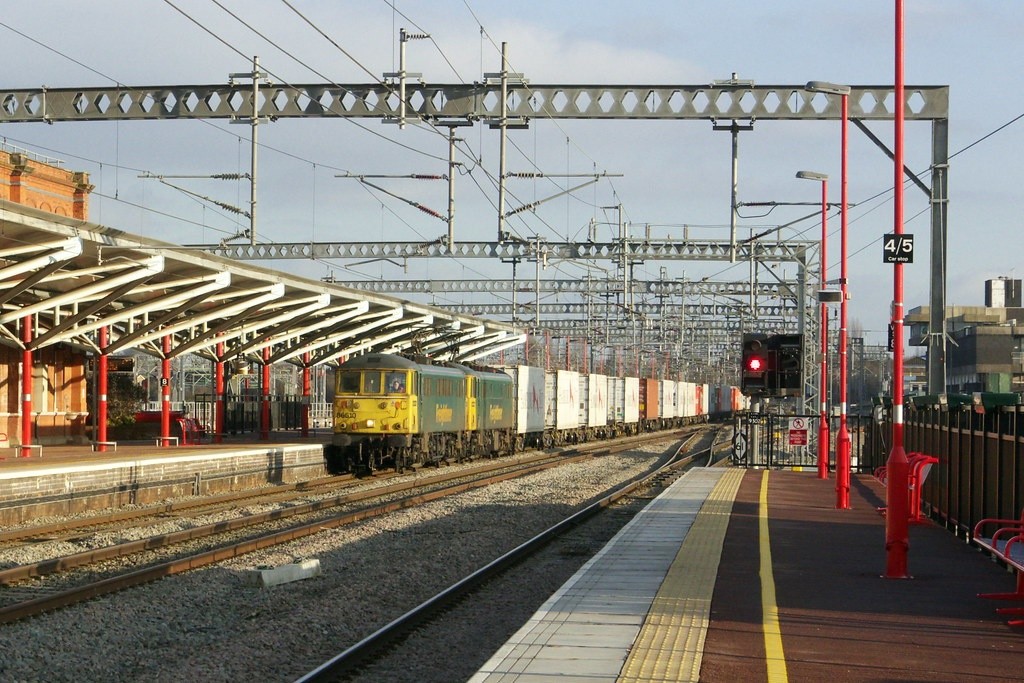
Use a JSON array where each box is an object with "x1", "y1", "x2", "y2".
[
  {"x1": 796, "y1": 170, "x2": 828, "y2": 480},
  {"x1": 803, "y1": 80, "x2": 854, "y2": 511}
]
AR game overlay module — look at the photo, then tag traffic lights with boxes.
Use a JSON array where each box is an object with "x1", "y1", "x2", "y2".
[{"x1": 742, "y1": 333, "x2": 772, "y2": 396}]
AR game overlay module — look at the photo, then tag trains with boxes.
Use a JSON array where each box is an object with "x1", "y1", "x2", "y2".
[{"x1": 332, "y1": 355, "x2": 763, "y2": 473}]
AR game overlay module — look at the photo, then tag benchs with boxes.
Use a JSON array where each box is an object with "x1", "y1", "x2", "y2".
[
  {"x1": 973, "y1": 511, "x2": 1023, "y2": 630},
  {"x1": 873, "y1": 451, "x2": 941, "y2": 526},
  {"x1": 177, "y1": 418, "x2": 212, "y2": 444}
]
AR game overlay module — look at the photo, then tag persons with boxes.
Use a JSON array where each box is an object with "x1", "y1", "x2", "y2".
[{"x1": 389, "y1": 381, "x2": 403, "y2": 393}]
